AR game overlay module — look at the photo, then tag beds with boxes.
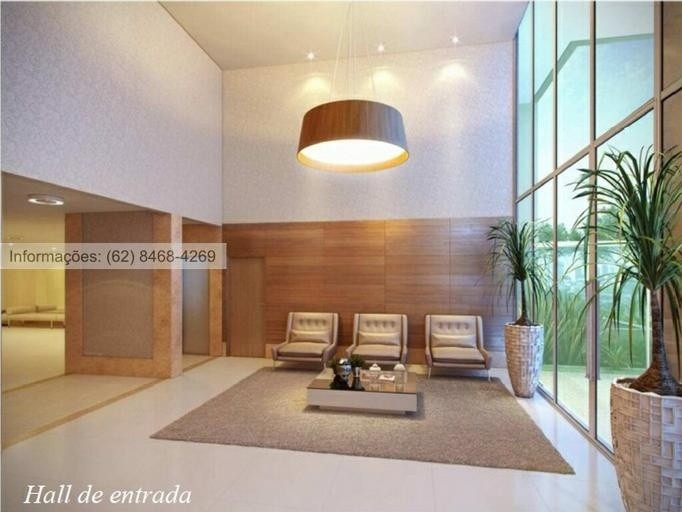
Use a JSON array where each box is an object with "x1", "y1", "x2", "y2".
[
  {"x1": 5, "y1": 303, "x2": 66, "y2": 327},
  {"x1": 306, "y1": 366, "x2": 418, "y2": 415}
]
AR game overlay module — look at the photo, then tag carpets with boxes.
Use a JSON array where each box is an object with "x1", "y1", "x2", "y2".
[{"x1": 149, "y1": 367, "x2": 576, "y2": 476}]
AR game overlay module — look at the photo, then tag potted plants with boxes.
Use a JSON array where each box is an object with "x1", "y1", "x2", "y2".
[
  {"x1": 348, "y1": 354, "x2": 364, "y2": 377},
  {"x1": 567, "y1": 144, "x2": 681, "y2": 512},
  {"x1": 478, "y1": 216, "x2": 562, "y2": 398}
]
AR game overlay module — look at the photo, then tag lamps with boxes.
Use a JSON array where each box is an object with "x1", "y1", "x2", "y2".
[{"x1": 298, "y1": 1, "x2": 410, "y2": 175}]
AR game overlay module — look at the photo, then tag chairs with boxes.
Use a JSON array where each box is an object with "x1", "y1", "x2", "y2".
[
  {"x1": 346, "y1": 313, "x2": 409, "y2": 366},
  {"x1": 424, "y1": 314, "x2": 492, "y2": 381},
  {"x1": 272, "y1": 310, "x2": 339, "y2": 369}
]
